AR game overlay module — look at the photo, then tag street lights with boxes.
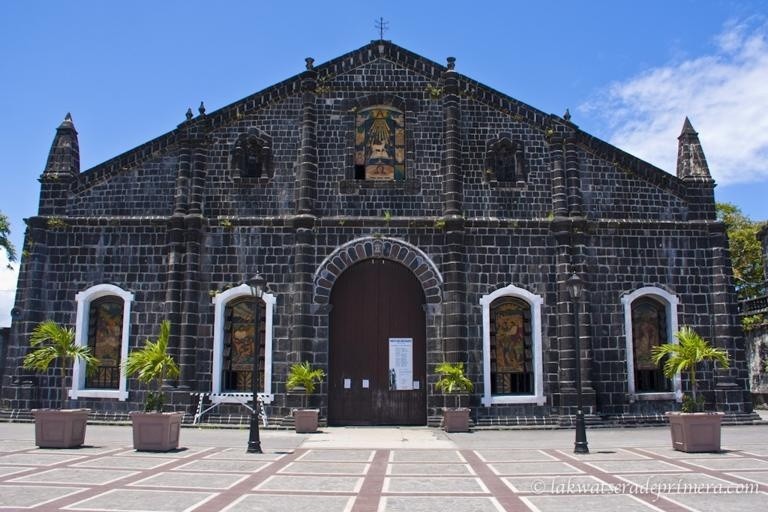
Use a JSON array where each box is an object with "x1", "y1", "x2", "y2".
[
  {"x1": 565, "y1": 270, "x2": 588, "y2": 454},
  {"x1": 246, "y1": 269, "x2": 268, "y2": 454}
]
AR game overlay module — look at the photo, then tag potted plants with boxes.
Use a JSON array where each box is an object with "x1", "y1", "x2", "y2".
[
  {"x1": 434, "y1": 362, "x2": 474, "y2": 432},
  {"x1": 23, "y1": 320, "x2": 102, "y2": 448},
  {"x1": 651, "y1": 324, "x2": 732, "y2": 452},
  {"x1": 119, "y1": 318, "x2": 186, "y2": 451},
  {"x1": 286, "y1": 360, "x2": 328, "y2": 433}
]
